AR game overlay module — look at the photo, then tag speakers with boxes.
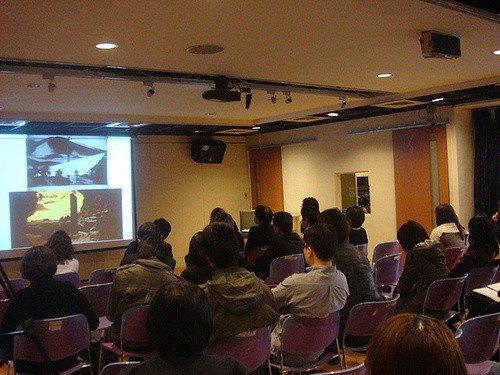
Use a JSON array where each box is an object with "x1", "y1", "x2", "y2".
[
  {"x1": 191, "y1": 139, "x2": 227, "y2": 163},
  {"x1": 422, "y1": 31, "x2": 462, "y2": 59}
]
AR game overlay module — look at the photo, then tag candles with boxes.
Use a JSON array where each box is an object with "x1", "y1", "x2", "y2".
[{"x1": 339, "y1": 96, "x2": 347, "y2": 109}]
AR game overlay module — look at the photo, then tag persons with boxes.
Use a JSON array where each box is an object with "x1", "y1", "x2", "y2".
[
  {"x1": 392, "y1": 204, "x2": 500, "y2": 311},
  {"x1": 44, "y1": 231, "x2": 80, "y2": 275},
  {"x1": 180, "y1": 197, "x2": 387, "y2": 375},
  {"x1": 0, "y1": 246, "x2": 100, "y2": 375},
  {"x1": 105, "y1": 218, "x2": 184, "y2": 354},
  {"x1": 123, "y1": 280, "x2": 247, "y2": 375},
  {"x1": 364, "y1": 313, "x2": 469, "y2": 375}
]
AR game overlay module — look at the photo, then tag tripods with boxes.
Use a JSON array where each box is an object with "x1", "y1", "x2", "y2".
[{"x1": 0, "y1": 262, "x2": 57, "y2": 375}]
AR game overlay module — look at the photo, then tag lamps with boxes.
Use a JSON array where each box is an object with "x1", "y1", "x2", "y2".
[
  {"x1": 245, "y1": 93, "x2": 252, "y2": 110},
  {"x1": 146, "y1": 89, "x2": 155, "y2": 97},
  {"x1": 283, "y1": 91, "x2": 292, "y2": 104},
  {"x1": 268, "y1": 94, "x2": 276, "y2": 104},
  {"x1": 48, "y1": 82, "x2": 56, "y2": 93}
]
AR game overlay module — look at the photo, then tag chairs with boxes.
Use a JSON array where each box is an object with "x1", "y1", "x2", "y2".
[{"x1": 0, "y1": 232, "x2": 500, "y2": 375}]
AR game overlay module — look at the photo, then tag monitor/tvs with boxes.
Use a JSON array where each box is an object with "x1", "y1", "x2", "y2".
[{"x1": 239, "y1": 209, "x2": 257, "y2": 233}]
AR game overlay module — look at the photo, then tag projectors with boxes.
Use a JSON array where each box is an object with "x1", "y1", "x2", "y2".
[{"x1": 201, "y1": 89, "x2": 242, "y2": 103}]
